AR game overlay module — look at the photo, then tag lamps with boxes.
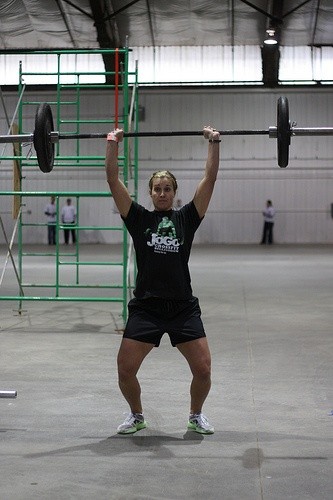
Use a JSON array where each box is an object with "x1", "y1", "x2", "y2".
[{"x1": 263, "y1": 19, "x2": 280, "y2": 47}]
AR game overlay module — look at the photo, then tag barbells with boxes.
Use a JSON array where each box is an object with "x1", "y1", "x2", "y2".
[{"x1": 0, "y1": 94, "x2": 333, "y2": 173}]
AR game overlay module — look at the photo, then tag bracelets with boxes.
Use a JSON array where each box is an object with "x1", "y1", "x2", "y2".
[
  {"x1": 209, "y1": 139, "x2": 221, "y2": 143},
  {"x1": 107, "y1": 136, "x2": 119, "y2": 142}
]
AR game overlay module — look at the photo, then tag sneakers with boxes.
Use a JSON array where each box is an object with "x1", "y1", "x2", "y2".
[
  {"x1": 116, "y1": 411, "x2": 147, "y2": 434},
  {"x1": 187, "y1": 411, "x2": 214, "y2": 434}
]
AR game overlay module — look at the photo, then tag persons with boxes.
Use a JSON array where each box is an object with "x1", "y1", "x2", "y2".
[
  {"x1": 106, "y1": 126, "x2": 221, "y2": 435},
  {"x1": 44, "y1": 197, "x2": 56, "y2": 244},
  {"x1": 260, "y1": 200, "x2": 275, "y2": 244},
  {"x1": 61, "y1": 198, "x2": 76, "y2": 245}
]
